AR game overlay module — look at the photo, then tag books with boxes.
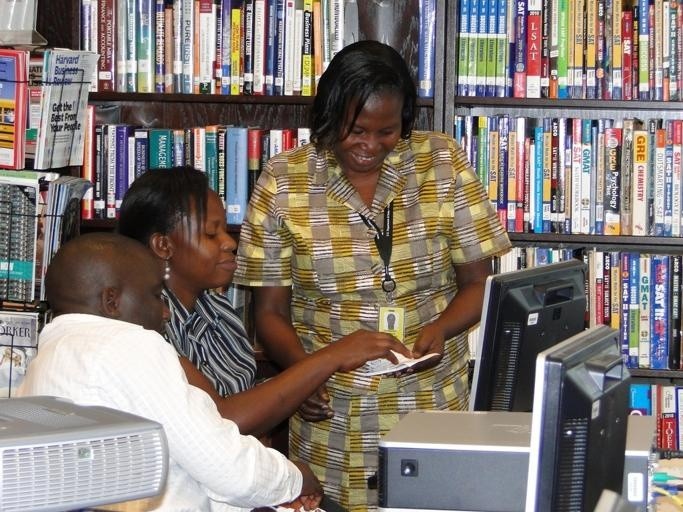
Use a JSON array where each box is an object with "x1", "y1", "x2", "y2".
[
  {"x1": 416, "y1": 0, "x2": 683, "y2": 455},
  {"x1": 0, "y1": 0, "x2": 346, "y2": 410}
]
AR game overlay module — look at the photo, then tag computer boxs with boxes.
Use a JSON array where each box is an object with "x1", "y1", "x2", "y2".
[
  {"x1": 0, "y1": 395, "x2": 169, "y2": 512},
  {"x1": 377, "y1": 411, "x2": 658, "y2": 512}
]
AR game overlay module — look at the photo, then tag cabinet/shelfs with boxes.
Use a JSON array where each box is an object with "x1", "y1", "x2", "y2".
[{"x1": 36, "y1": 0, "x2": 683, "y2": 384}]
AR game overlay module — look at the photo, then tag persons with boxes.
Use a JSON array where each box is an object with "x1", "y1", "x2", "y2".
[
  {"x1": 9, "y1": 234, "x2": 326, "y2": 511},
  {"x1": 117, "y1": 162, "x2": 415, "y2": 445},
  {"x1": 383, "y1": 313, "x2": 396, "y2": 330},
  {"x1": 230, "y1": 42, "x2": 513, "y2": 511}
]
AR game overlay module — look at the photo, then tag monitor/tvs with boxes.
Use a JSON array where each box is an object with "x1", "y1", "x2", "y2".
[
  {"x1": 468, "y1": 259, "x2": 587, "y2": 412},
  {"x1": 525, "y1": 323, "x2": 632, "y2": 512}
]
